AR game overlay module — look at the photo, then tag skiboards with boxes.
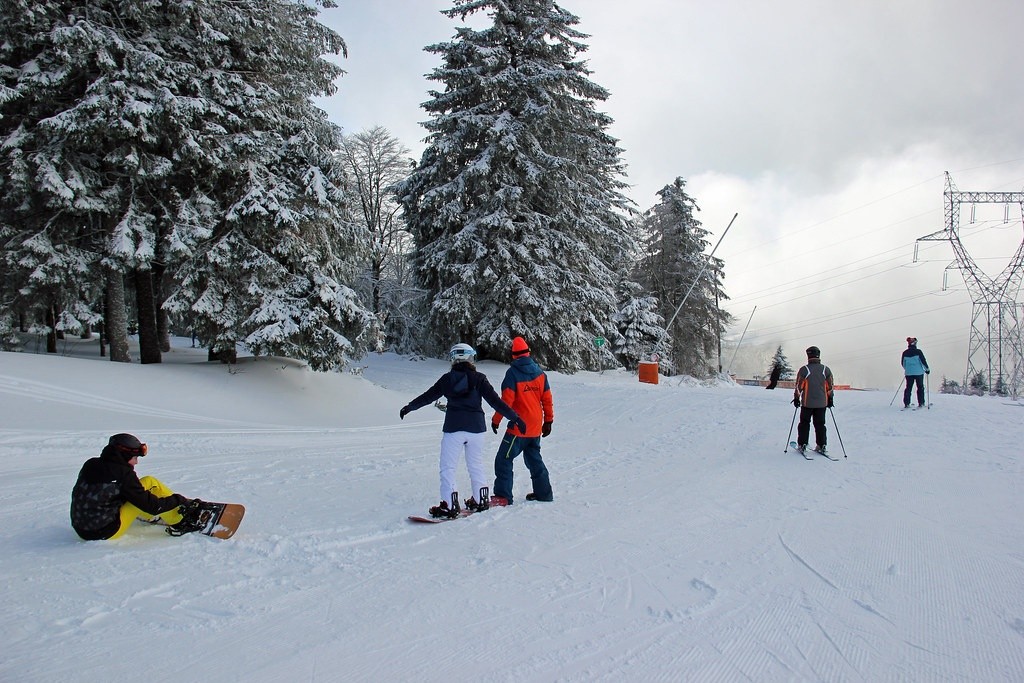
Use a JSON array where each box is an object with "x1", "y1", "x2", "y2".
[
  {"x1": 900, "y1": 403, "x2": 933, "y2": 411},
  {"x1": 790, "y1": 440, "x2": 839, "y2": 461}
]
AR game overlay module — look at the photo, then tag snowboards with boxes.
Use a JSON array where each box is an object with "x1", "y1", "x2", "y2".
[
  {"x1": 408, "y1": 496, "x2": 508, "y2": 524},
  {"x1": 136, "y1": 495, "x2": 246, "y2": 539}
]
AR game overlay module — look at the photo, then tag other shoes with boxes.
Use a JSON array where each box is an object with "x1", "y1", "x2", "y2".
[
  {"x1": 490, "y1": 496, "x2": 513, "y2": 506},
  {"x1": 466, "y1": 499, "x2": 477, "y2": 509},
  {"x1": 814, "y1": 445, "x2": 826, "y2": 452},
  {"x1": 429, "y1": 503, "x2": 449, "y2": 516},
  {"x1": 175, "y1": 521, "x2": 198, "y2": 532},
  {"x1": 904, "y1": 401, "x2": 910, "y2": 406},
  {"x1": 527, "y1": 492, "x2": 537, "y2": 501},
  {"x1": 919, "y1": 400, "x2": 925, "y2": 406},
  {"x1": 797, "y1": 444, "x2": 807, "y2": 451}
]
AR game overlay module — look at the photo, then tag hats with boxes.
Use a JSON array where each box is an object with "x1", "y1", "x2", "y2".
[
  {"x1": 107, "y1": 434, "x2": 142, "y2": 462},
  {"x1": 450, "y1": 344, "x2": 477, "y2": 366},
  {"x1": 806, "y1": 347, "x2": 821, "y2": 359},
  {"x1": 512, "y1": 337, "x2": 530, "y2": 359},
  {"x1": 907, "y1": 337, "x2": 916, "y2": 345}
]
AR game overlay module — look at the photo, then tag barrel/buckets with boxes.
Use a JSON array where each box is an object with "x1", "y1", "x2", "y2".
[{"x1": 639, "y1": 361, "x2": 658, "y2": 384}]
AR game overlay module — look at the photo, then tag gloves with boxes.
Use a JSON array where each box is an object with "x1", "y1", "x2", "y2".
[
  {"x1": 790, "y1": 399, "x2": 801, "y2": 408},
  {"x1": 827, "y1": 397, "x2": 835, "y2": 408},
  {"x1": 400, "y1": 405, "x2": 409, "y2": 420},
  {"x1": 515, "y1": 417, "x2": 526, "y2": 435},
  {"x1": 925, "y1": 370, "x2": 930, "y2": 375},
  {"x1": 541, "y1": 422, "x2": 552, "y2": 437},
  {"x1": 492, "y1": 422, "x2": 499, "y2": 434}
]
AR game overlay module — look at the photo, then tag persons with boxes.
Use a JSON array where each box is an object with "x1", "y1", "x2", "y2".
[
  {"x1": 399, "y1": 344, "x2": 526, "y2": 518},
  {"x1": 71, "y1": 433, "x2": 208, "y2": 540},
  {"x1": 794, "y1": 346, "x2": 834, "y2": 452},
  {"x1": 901, "y1": 337, "x2": 930, "y2": 408},
  {"x1": 766, "y1": 362, "x2": 782, "y2": 390},
  {"x1": 491, "y1": 337, "x2": 554, "y2": 504}
]
